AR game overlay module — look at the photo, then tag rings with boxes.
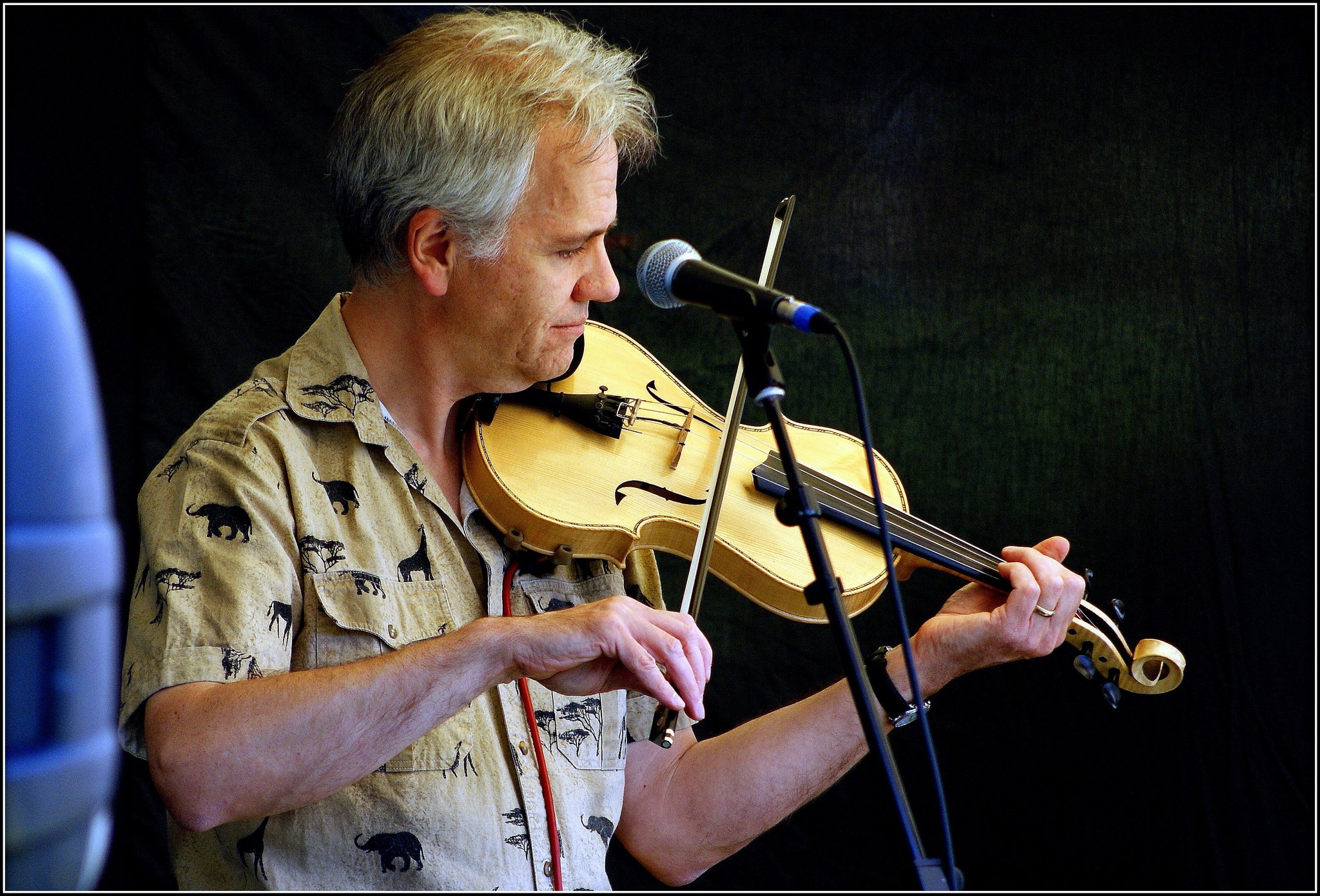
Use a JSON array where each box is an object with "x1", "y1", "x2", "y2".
[{"x1": 1034, "y1": 606, "x2": 1057, "y2": 618}]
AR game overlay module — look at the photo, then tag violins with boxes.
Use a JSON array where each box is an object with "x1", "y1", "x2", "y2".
[{"x1": 466, "y1": 316, "x2": 1186, "y2": 696}]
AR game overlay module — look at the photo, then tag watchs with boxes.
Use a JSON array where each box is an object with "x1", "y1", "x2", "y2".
[{"x1": 867, "y1": 646, "x2": 930, "y2": 727}]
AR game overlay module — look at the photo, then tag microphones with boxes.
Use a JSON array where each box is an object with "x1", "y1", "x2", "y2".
[{"x1": 636, "y1": 239, "x2": 842, "y2": 338}]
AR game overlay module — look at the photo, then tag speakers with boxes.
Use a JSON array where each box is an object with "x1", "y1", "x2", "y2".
[{"x1": 3, "y1": 234, "x2": 124, "y2": 896}]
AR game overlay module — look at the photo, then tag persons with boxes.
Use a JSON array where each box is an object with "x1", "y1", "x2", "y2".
[{"x1": 114, "y1": 13, "x2": 1087, "y2": 891}]
